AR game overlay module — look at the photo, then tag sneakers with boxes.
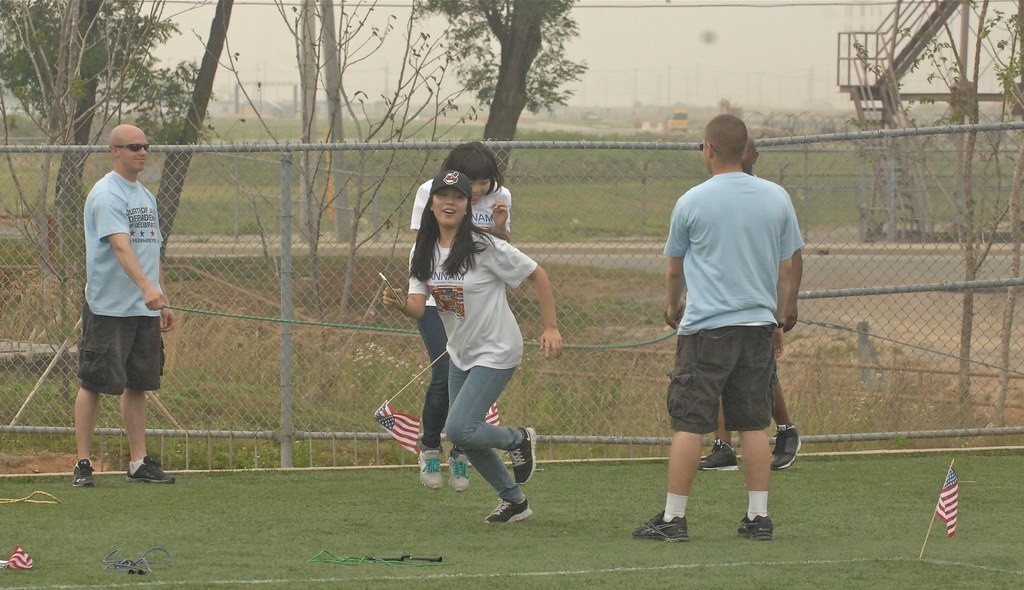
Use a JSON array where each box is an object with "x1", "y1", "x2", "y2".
[
  {"x1": 738, "y1": 511, "x2": 773, "y2": 540},
  {"x1": 770, "y1": 423, "x2": 802, "y2": 470},
  {"x1": 632, "y1": 510, "x2": 689, "y2": 543},
  {"x1": 418, "y1": 448, "x2": 444, "y2": 490},
  {"x1": 698, "y1": 436, "x2": 740, "y2": 471},
  {"x1": 483, "y1": 493, "x2": 532, "y2": 524},
  {"x1": 125, "y1": 456, "x2": 175, "y2": 484},
  {"x1": 447, "y1": 448, "x2": 470, "y2": 491},
  {"x1": 507, "y1": 427, "x2": 536, "y2": 484},
  {"x1": 72, "y1": 458, "x2": 97, "y2": 487}
]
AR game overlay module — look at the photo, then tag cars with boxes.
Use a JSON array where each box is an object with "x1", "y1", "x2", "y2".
[{"x1": 671, "y1": 109, "x2": 688, "y2": 132}]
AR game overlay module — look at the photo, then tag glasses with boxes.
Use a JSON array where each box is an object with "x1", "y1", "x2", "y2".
[
  {"x1": 699, "y1": 143, "x2": 715, "y2": 151},
  {"x1": 115, "y1": 143, "x2": 150, "y2": 152}
]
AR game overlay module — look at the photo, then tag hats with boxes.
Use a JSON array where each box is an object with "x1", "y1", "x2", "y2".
[{"x1": 430, "y1": 169, "x2": 473, "y2": 199}]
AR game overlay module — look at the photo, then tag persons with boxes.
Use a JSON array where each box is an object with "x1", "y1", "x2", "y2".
[
  {"x1": 407, "y1": 143, "x2": 511, "y2": 492},
  {"x1": 633, "y1": 113, "x2": 804, "y2": 543},
  {"x1": 73, "y1": 125, "x2": 177, "y2": 487},
  {"x1": 696, "y1": 134, "x2": 804, "y2": 471},
  {"x1": 384, "y1": 142, "x2": 564, "y2": 522}
]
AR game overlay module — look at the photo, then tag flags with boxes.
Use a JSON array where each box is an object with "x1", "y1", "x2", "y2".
[
  {"x1": 374, "y1": 402, "x2": 423, "y2": 454},
  {"x1": 936, "y1": 467, "x2": 959, "y2": 538},
  {"x1": 484, "y1": 401, "x2": 500, "y2": 427}
]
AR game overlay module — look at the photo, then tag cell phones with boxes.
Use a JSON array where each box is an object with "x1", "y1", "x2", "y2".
[{"x1": 378, "y1": 273, "x2": 403, "y2": 305}]
